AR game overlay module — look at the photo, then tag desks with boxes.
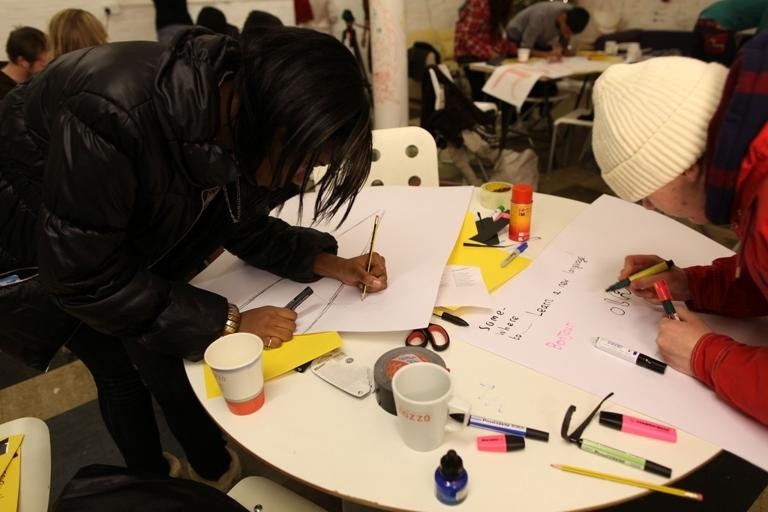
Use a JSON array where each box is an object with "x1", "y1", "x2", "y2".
[{"x1": 183, "y1": 185, "x2": 725, "y2": 512}]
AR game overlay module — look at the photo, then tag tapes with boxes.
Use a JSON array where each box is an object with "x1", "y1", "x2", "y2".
[{"x1": 373, "y1": 346, "x2": 447, "y2": 416}]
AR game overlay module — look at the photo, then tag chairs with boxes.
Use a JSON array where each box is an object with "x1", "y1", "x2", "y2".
[
  {"x1": 311, "y1": 124, "x2": 440, "y2": 188},
  {"x1": 407, "y1": 35, "x2": 641, "y2": 182}
]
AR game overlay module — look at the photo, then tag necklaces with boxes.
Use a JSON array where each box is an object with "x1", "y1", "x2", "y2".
[{"x1": 223, "y1": 175, "x2": 241, "y2": 224}]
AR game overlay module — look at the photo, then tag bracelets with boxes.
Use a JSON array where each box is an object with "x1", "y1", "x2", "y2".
[{"x1": 223, "y1": 302, "x2": 240, "y2": 335}]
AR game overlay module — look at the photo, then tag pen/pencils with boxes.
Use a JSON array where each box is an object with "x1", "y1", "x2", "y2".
[
  {"x1": 431, "y1": 310, "x2": 469, "y2": 327},
  {"x1": 569, "y1": 438, "x2": 672, "y2": 478},
  {"x1": 550, "y1": 463, "x2": 704, "y2": 501},
  {"x1": 501, "y1": 242, "x2": 529, "y2": 268},
  {"x1": 606, "y1": 260, "x2": 674, "y2": 294},
  {"x1": 285, "y1": 286, "x2": 314, "y2": 311},
  {"x1": 449, "y1": 413, "x2": 549, "y2": 441},
  {"x1": 360, "y1": 214, "x2": 379, "y2": 301}
]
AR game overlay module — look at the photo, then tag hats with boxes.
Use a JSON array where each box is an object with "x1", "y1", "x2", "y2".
[{"x1": 590, "y1": 56, "x2": 730, "y2": 202}]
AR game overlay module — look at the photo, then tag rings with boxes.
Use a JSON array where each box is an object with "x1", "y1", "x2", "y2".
[{"x1": 268, "y1": 336, "x2": 272, "y2": 346}]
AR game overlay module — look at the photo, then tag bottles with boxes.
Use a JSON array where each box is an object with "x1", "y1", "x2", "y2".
[
  {"x1": 434, "y1": 450, "x2": 469, "y2": 506},
  {"x1": 510, "y1": 182, "x2": 534, "y2": 245}
]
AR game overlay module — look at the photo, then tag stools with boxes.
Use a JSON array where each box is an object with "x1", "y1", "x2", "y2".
[
  {"x1": 0, "y1": 414, "x2": 54, "y2": 512},
  {"x1": 226, "y1": 474, "x2": 328, "y2": 512}
]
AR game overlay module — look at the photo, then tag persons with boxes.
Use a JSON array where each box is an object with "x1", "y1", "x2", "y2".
[
  {"x1": 452, "y1": 0, "x2": 517, "y2": 102},
  {"x1": 504, "y1": 1, "x2": 590, "y2": 120},
  {"x1": 694, "y1": 0, "x2": 768, "y2": 67},
  {"x1": 0, "y1": 26, "x2": 388, "y2": 493},
  {"x1": 591, "y1": 32, "x2": 768, "y2": 426},
  {"x1": 0, "y1": 27, "x2": 47, "y2": 100},
  {"x1": 48, "y1": 8, "x2": 109, "y2": 58}
]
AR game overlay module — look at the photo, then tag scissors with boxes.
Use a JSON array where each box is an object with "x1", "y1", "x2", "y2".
[{"x1": 406, "y1": 323, "x2": 449, "y2": 351}]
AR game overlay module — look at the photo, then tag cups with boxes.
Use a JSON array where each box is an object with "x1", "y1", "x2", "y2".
[
  {"x1": 202, "y1": 331, "x2": 268, "y2": 415},
  {"x1": 516, "y1": 48, "x2": 530, "y2": 62},
  {"x1": 393, "y1": 361, "x2": 455, "y2": 449},
  {"x1": 604, "y1": 41, "x2": 618, "y2": 56},
  {"x1": 627, "y1": 42, "x2": 641, "y2": 64}
]
